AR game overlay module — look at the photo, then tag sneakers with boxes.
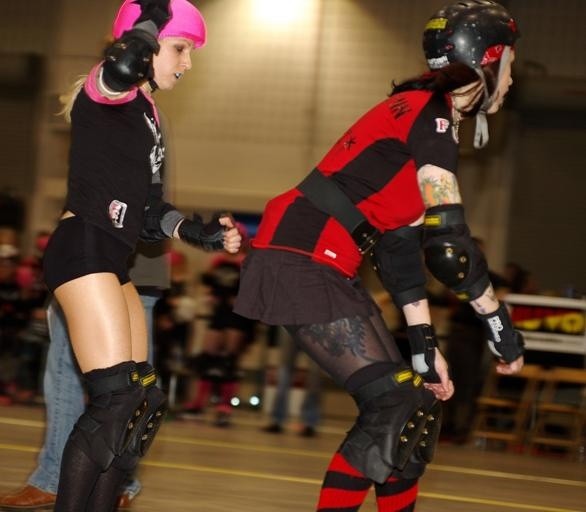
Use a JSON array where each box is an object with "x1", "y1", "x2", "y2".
[
  {"x1": 182, "y1": 403, "x2": 233, "y2": 425},
  {"x1": 262, "y1": 423, "x2": 317, "y2": 436}
]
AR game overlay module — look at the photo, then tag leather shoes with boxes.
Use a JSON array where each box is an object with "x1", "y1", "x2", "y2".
[{"x1": 0, "y1": 484, "x2": 57, "y2": 511}]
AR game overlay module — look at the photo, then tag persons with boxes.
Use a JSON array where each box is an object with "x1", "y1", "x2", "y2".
[
  {"x1": 232, "y1": 2, "x2": 527, "y2": 512},
  {"x1": 3, "y1": 183, "x2": 582, "y2": 462},
  {"x1": 0, "y1": 97, "x2": 176, "y2": 512},
  {"x1": 40, "y1": 0, "x2": 244, "y2": 512}
]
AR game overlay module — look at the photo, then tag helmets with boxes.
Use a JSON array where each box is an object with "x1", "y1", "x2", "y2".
[
  {"x1": 112, "y1": 0, "x2": 206, "y2": 48},
  {"x1": 423, "y1": 0, "x2": 519, "y2": 70}
]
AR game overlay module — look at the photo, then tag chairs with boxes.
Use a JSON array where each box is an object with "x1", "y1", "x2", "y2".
[{"x1": 471, "y1": 363, "x2": 585, "y2": 461}]
